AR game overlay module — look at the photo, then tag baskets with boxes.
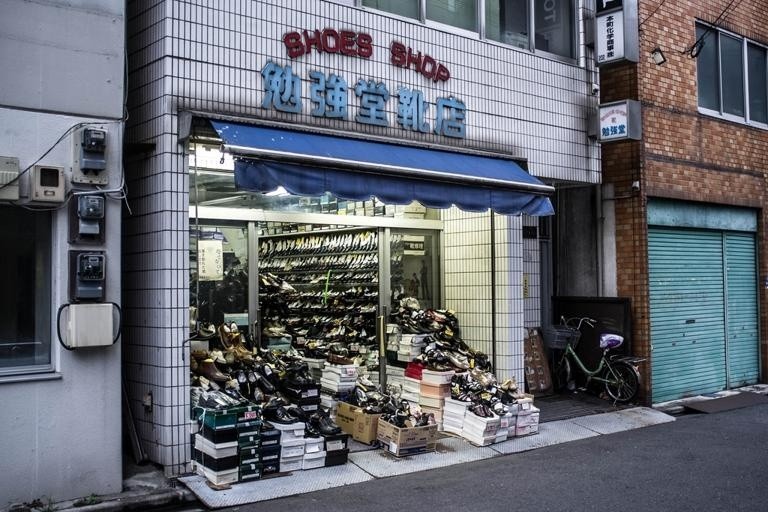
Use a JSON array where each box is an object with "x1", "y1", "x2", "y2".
[{"x1": 544, "y1": 325, "x2": 581, "y2": 349}]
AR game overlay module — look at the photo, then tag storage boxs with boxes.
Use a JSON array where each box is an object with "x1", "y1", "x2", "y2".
[
  {"x1": 265, "y1": 323, "x2": 547, "y2": 457},
  {"x1": 193, "y1": 394, "x2": 350, "y2": 488},
  {"x1": 255, "y1": 194, "x2": 440, "y2": 228},
  {"x1": 224, "y1": 311, "x2": 254, "y2": 328},
  {"x1": 186, "y1": 223, "x2": 239, "y2": 256}
]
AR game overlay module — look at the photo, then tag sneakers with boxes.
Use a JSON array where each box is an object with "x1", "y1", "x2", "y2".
[
  {"x1": 391, "y1": 302, "x2": 526, "y2": 418},
  {"x1": 190, "y1": 323, "x2": 342, "y2": 437},
  {"x1": 192, "y1": 231, "x2": 378, "y2": 364},
  {"x1": 351, "y1": 381, "x2": 436, "y2": 426}
]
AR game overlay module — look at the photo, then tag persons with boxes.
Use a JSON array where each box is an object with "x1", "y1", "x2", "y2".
[{"x1": 412, "y1": 260, "x2": 430, "y2": 300}]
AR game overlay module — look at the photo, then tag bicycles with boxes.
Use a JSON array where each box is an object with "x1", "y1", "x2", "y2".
[{"x1": 545, "y1": 316, "x2": 647, "y2": 407}]
